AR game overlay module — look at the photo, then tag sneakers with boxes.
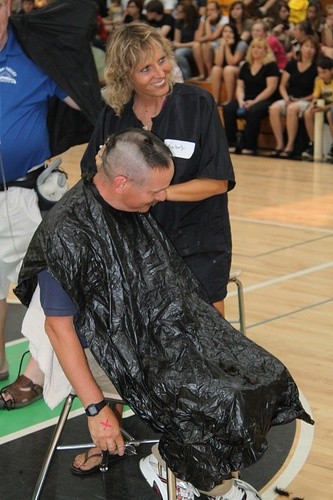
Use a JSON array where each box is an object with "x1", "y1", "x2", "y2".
[
  {"x1": 207, "y1": 478, "x2": 262, "y2": 500},
  {"x1": 139, "y1": 453, "x2": 201, "y2": 500}
]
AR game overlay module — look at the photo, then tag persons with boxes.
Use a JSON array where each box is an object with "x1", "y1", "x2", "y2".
[
  {"x1": 0, "y1": 0, "x2": 83, "y2": 409},
  {"x1": 12, "y1": 0, "x2": 333, "y2": 160},
  {"x1": 13, "y1": 128, "x2": 316, "y2": 500},
  {"x1": 71, "y1": 21, "x2": 235, "y2": 475}
]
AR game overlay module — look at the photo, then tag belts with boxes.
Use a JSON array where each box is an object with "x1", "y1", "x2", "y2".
[{"x1": 7, "y1": 164, "x2": 48, "y2": 187}]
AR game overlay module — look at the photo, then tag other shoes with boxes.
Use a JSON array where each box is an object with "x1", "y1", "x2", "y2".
[{"x1": 227, "y1": 141, "x2": 333, "y2": 162}]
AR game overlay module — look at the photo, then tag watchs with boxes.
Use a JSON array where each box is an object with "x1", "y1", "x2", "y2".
[{"x1": 85, "y1": 398, "x2": 109, "y2": 416}]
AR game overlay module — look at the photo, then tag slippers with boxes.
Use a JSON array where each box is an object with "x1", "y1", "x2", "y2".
[
  {"x1": 0, "y1": 375, "x2": 43, "y2": 408},
  {"x1": 71, "y1": 447, "x2": 127, "y2": 476}
]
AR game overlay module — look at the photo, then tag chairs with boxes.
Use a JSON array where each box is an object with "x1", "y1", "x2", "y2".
[{"x1": 31, "y1": 393, "x2": 177, "y2": 500}]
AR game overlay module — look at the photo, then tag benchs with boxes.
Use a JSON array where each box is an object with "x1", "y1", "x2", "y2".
[{"x1": 186, "y1": 80, "x2": 313, "y2": 157}]
